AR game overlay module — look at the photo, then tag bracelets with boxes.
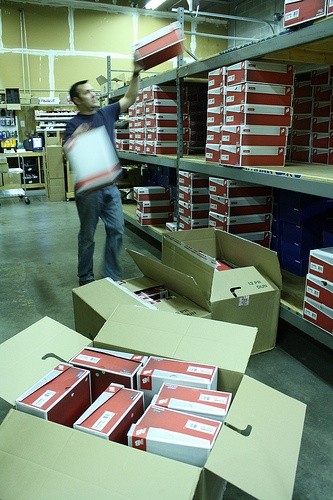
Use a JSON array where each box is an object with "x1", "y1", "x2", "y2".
[{"x1": 134, "y1": 72, "x2": 139, "y2": 76}]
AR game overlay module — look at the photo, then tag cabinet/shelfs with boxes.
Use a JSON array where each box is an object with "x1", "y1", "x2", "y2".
[{"x1": 107, "y1": 7, "x2": 333, "y2": 348}]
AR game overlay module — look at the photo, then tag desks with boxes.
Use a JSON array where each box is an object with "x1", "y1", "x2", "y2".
[{"x1": 5, "y1": 153, "x2": 52, "y2": 188}]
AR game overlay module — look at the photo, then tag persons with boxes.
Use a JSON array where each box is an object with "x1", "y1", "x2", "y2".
[{"x1": 63, "y1": 58, "x2": 146, "y2": 286}]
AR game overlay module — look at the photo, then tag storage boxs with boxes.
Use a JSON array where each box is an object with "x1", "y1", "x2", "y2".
[
  {"x1": 45, "y1": 137, "x2": 65, "y2": 201},
  {"x1": 113, "y1": 0, "x2": 332, "y2": 339},
  {"x1": 0, "y1": 228, "x2": 307, "y2": 500},
  {"x1": 0, "y1": 156, "x2": 22, "y2": 189}
]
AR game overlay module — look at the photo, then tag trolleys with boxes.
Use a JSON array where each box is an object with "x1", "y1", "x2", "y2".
[{"x1": 0, "y1": 152, "x2": 31, "y2": 205}]
